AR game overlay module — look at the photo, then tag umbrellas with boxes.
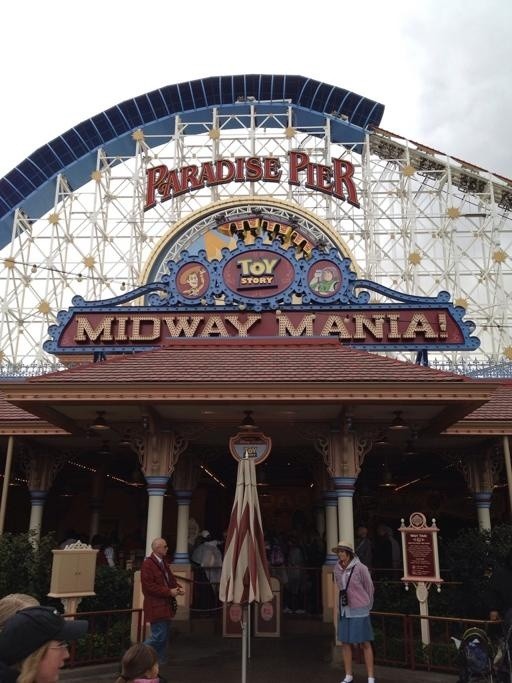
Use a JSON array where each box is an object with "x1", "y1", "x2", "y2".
[{"x1": 217, "y1": 449, "x2": 276, "y2": 681}]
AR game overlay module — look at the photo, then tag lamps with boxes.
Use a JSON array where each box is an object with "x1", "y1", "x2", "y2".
[
  {"x1": 403, "y1": 440, "x2": 419, "y2": 456},
  {"x1": 96, "y1": 440, "x2": 112, "y2": 456},
  {"x1": 238, "y1": 411, "x2": 260, "y2": 429},
  {"x1": 389, "y1": 411, "x2": 409, "y2": 430},
  {"x1": 89, "y1": 411, "x2": 111, "y2": 431}
]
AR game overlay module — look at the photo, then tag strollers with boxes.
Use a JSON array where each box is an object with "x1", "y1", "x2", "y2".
[{"x1": 457, "y1": 625, "x2": 497, "y2": 683}]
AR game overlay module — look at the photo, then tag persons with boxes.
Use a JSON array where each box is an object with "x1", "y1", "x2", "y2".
[
  {"x1": 58, "y1": 527, "x2": 146, "y2": 572},
  {"x1": 137, "y1": 535, "x2": 187, "y2": 669},
  {"x1": 189, "y1": 527, "x2": 228, "y2": 584},
  {"x1": 264, "y1": 532, "x2": 327, "y2": 616},
  {"x1": 355, "y1": 526, "x2": 402, "y2": 605},
  {"x1": 0, "y1": 589, "x2": 44, "y2": 640},
  {"x1": 112, "y1": 642, "x2": 170, "y2": 682},
  {"x1": 486, "y1": 546, "x2": 511, "y2": 682},
  {"x1": 330, "y1": 540, "x2": 378, "y2": 682},
  {"x1": 0, "y1": 604, "x2": 89, "y2": 682}
]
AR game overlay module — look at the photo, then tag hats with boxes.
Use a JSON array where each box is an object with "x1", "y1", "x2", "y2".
[
  {"x1": 0, "y1": 607, "x2": 88, "y2": 665},
  {"x1": 332, "y1": 541, "x2": 354, "y2": 553}
]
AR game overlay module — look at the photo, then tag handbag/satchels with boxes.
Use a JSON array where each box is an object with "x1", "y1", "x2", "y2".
[{"x1": 339, "y1": 590, "x2": 347, "y2": 606}]
